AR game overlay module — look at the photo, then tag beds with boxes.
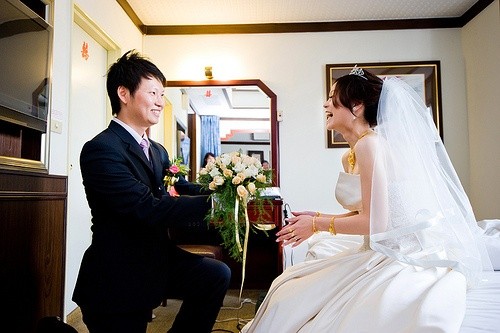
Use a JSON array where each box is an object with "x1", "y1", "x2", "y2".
[{"x1": 308, "y1": 218, "x2": 500, "y2": 333}]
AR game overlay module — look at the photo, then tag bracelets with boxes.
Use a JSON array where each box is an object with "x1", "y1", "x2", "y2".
[
  {"x1": 329, "y1": 216, "x2": 337, "y2": 235},
  {"x1": 316, "y1": 210, "x2": 322, "y2": 218},
  {"x1": 312, "y1": 216, "x2": 320, "y2": 234}
]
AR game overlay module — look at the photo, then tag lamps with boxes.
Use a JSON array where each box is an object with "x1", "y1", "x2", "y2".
[{"x1": 205, "y1": 66, "x2": 213, "y2": 79}]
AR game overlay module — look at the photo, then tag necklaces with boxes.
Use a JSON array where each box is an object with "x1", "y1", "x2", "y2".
[{"x1": 348, "y1": 130, "x2": 374, "y2": 170}]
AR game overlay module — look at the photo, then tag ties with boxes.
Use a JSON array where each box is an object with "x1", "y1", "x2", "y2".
[{"x1": 140, "y1": 138, "x2": 150, "y2": 161}]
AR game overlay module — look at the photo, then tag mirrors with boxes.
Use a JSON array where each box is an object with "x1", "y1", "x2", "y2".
[{"x1": 145, "y1": 80, "x2": 280, "y2": 187}]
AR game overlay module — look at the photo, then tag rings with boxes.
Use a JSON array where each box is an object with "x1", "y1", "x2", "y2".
[
  {"x1": 290, "y1": 232, "x2": 294, "y2": 237},
  {"x1": 293, "y1": 237, "x2": 298, "y2": 241},
  {"x1": 288, "y1": 226, "x2": 293, "y2": 232}
]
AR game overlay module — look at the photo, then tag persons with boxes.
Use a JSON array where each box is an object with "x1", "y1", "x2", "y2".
[
  {"x1": 202, "y1": 152, "x2": 217, "y2": 168},
  {"x1": 29, "y1": 49, "x2": 259, "y2": 333},
  {"x1": 237, "y1": 64, "x2": 494, "y2": 332},
  {"x1": 261, "y1": 160, "x2": 272, "y2": 171}
]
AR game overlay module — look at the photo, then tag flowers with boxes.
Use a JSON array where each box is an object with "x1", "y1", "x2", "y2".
[
  {"x1": 162, "y1": 154, "x2": 191, "y2": 197},
  {"x1": 192, "y1": 150, "x2": 275, "y2": 304}
]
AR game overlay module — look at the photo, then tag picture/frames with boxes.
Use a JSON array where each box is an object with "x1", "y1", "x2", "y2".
[
  {"x1": 248, "y1": 151, "x2": 263, "y2": 166},
  {"x1": 326, "y1": 60, "x2": 444, "y2": 148}
]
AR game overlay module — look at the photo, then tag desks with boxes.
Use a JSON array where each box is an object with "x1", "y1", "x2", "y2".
[
  {"x1": 207, "y1": 196, "x2": 283, "y2": 276},
  {"x1": 0, "y1": 169, "x2": 68, "y2": 333}
]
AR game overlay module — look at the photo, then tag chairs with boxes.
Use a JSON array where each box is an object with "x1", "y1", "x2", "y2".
[{"x1": 162, "y1": 220, "x2": 226, "y2": 306}]
